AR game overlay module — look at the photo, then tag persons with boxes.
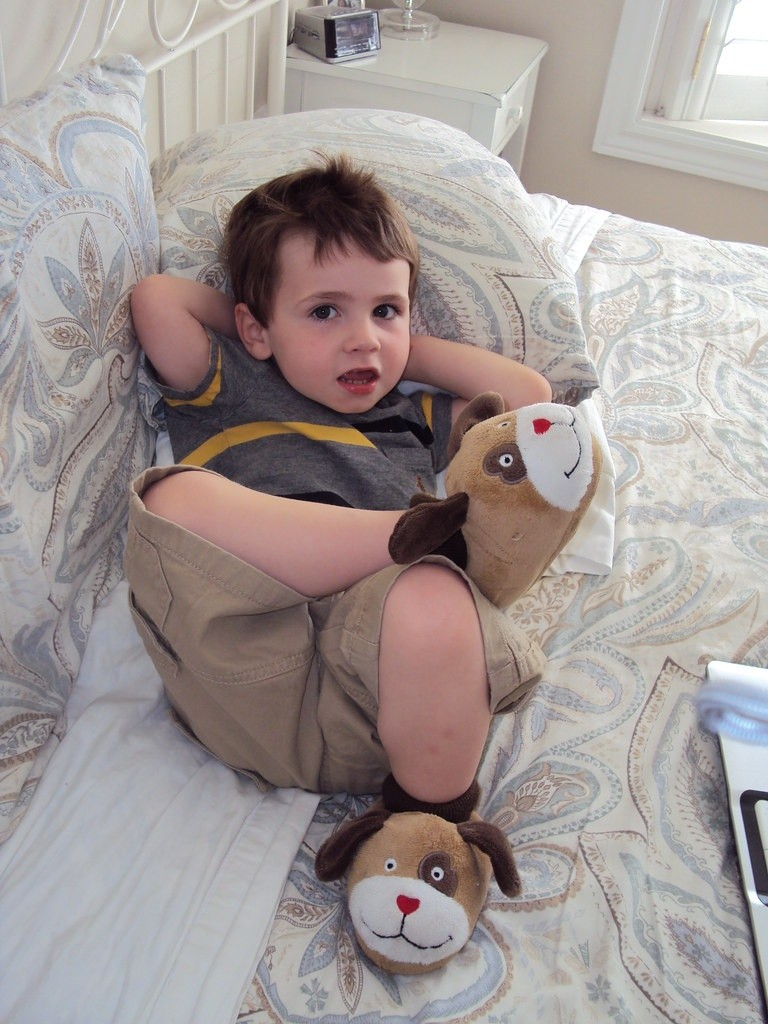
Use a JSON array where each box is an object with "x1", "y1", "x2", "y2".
[{"x1": 126, "y1": 152, "x2": 601, "y2": 974}]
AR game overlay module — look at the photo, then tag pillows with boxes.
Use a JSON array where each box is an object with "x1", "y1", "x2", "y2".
[
  {"x1": 137, "y1": 107, "x2": 597, "y2": 427},
  {"x1": 0, "y1": 46, "x2": 155, "y2": 851}
]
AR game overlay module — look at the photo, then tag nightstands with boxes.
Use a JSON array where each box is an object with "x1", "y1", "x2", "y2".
[{"x1": 285, "y1": 21, "x2": 549, "y2": 181}]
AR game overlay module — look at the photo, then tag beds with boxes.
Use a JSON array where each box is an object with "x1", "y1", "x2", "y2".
[{"x1": 0, "y1": 0, "x2": 768, "y2": 1024}]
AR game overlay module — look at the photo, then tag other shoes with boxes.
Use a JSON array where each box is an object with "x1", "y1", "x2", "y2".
[
  {"x1": 315, "y1": 801, "x2": 521, "y2": 975},
  {"x1": 389, "y1": 391, "x2": 602, "y2": 613}
]
